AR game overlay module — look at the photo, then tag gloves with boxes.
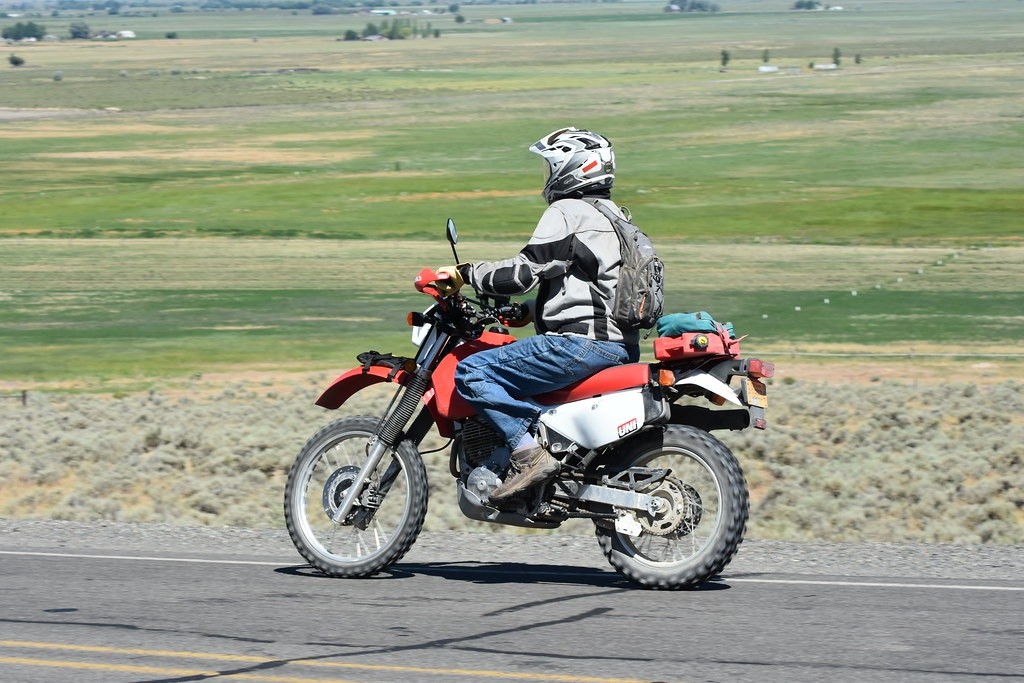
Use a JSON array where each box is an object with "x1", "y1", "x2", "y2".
[
  {"x1": 503, "y1": 301, "x2": 532, "y2": 327},
  {"x1": 435, "y1": 262, "x2": 471, "y2": 297}
]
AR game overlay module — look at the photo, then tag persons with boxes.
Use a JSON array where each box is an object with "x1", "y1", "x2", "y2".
[{"x1": 434, "y1": 127, "x2": 639, "y2": 503}]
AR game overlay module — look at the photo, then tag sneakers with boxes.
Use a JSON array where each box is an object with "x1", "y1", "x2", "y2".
[{"x1": 488, "y1": 445, "x2": 561, "y2": 506}]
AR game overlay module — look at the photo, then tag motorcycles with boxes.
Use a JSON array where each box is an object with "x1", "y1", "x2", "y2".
[{"x1": 283, "y1": 217, "x2": 775, "y2": 591}]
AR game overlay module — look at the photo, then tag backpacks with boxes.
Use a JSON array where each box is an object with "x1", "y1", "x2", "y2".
[{"x1": 580, "y1": 197, "x2": 664, "y2": 340}]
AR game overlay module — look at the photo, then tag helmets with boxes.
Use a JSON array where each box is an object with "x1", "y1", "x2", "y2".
[{"x1": 528, "y1": 126, "x2": 616, "y2": 205}]
